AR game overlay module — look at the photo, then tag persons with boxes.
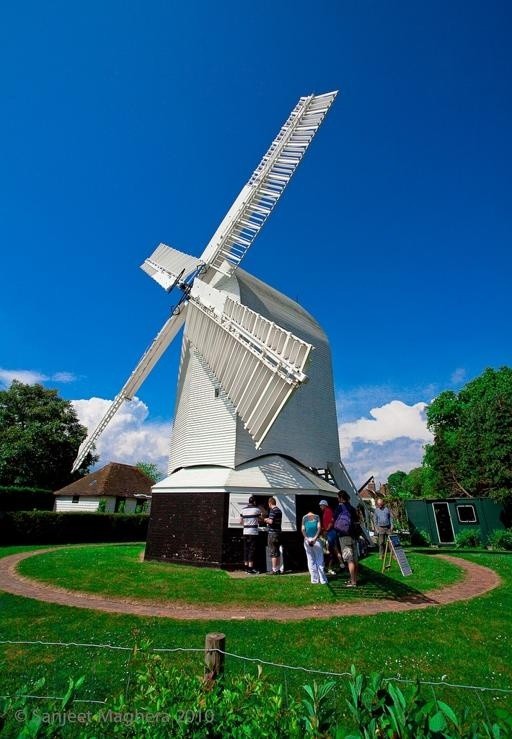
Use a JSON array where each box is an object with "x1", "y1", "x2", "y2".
[
  {"x1": 264, "y1": 498, "x2": 282, "y2": 574},
  {"x1": 334, "y1": 491, "x2": 359, "y2": 586},
  {"x1": 372, "y1": 498, "x2": 398, "y2": 561},
  {"x1": 300, "y1": 510, "x2": 329, "y2": 584},
  {"x1": 238, "y1": 496, "x2": 264, "y2": 573},
  {"x1": 318, "y1": 499, "x2": 345, "y2": 574}
]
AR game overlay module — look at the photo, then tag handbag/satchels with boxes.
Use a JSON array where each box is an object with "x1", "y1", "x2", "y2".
[{"x1": 334, "y1": 511, "x2": 351, "y2": 532}]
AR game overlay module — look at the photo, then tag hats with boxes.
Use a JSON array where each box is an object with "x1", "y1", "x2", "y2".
[{"x1": 320, "y1": 500, "x2": 328, "y2": 506}]
